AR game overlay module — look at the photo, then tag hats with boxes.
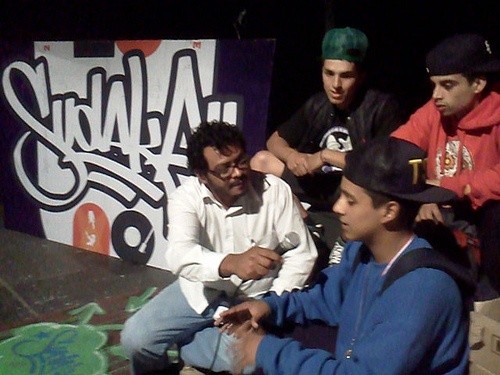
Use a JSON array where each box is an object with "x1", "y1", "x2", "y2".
[
  {"x1": 426, "y1": 33, "x2": 500, "y2": 77},
  {"x1": 342, "y1": 137, "x2": 457, "y2": 204},
  {"x1": 320, "y1": 27, "x2": 369, "y2": 64}
]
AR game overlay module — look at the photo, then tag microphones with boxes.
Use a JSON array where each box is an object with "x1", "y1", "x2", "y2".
[{"x1": 241, "y1": 232, "x2": 300, "y2": 283}]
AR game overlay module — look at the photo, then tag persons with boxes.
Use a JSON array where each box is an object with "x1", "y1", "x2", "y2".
[
  {"x1": 214, "y1": 136, "x2": 471, "y2": 375},
  {"x1": 390, "y1": 33, "x2": 500, "y2": 314},
  {"x1": 120, "y1": 120, "x2": 318, "y2": 375},
  {"x1": 250, "y1": 26, "x2": 408, "y2": 267}
]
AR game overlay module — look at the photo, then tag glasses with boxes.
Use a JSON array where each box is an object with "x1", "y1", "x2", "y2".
[{"x1": 209, "y1": 154, "x2": 250, "y2": 181}]
